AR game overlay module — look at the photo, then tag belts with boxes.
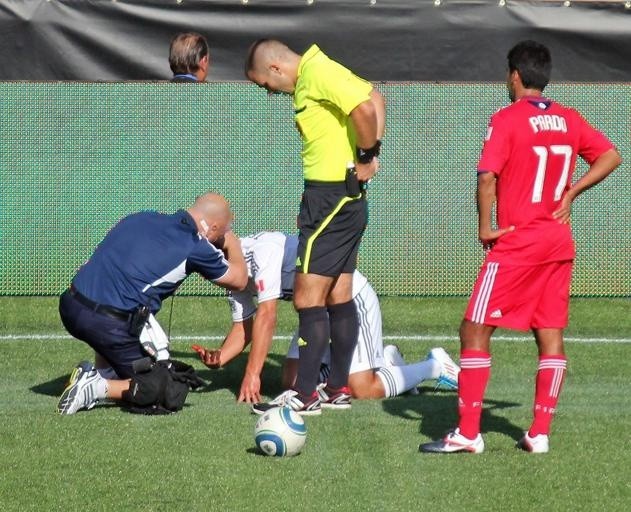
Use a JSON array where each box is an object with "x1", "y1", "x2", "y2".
[{"x1": 68, "y1": 282, "x2": 130, "y2": 322}]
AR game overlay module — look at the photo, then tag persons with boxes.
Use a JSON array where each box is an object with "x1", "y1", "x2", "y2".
[
  {"x1": 244, "y1": 38, "x2": 388, "y2": 418},
  {"x1": 169, "y1": 29, "x2": 210, "y2": 81},
  {"x1": 192, "y1": 230, "x2": 461, "y2": 399},
  {"x1": 420, "y1": 41, "x2": 623, "y2": 454},
  {"x1": 55, "y1": 192, "x2": 248, "y2": 416}
]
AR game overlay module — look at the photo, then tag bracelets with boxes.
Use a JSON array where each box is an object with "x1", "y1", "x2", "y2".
[
  {"x1": 376, "y1": 140, "x2": 381, "y2": 156},
  {"x1": 353, "y1": 140, "x2": 377, "y2": 165}
]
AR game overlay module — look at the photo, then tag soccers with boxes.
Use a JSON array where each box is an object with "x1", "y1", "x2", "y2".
[{"x1": 256, "y1": 408, "x2": 307, "y2": 456}]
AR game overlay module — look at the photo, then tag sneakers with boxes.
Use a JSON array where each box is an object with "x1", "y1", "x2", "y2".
[
  {"x1": 429, "y1": 347, "x2": 462, "y2": 391},
  {"x1": 55, "y1": 360, "x2": 102, "y2": 416},
  {"x1": 418, "y1": 427, "x2": 485, "y2": 455},
  {"x1": 383, "y1": 345, "x2": 418, "y2": 396},
  {"x1": 252, "y1": 386, "x2": 321, "y2": 416},
  {"x1": 515, "y1": 432, "x2": 548, "y2": 453},
  {"x1": 97, "y1": 365, "x2": 118, "y2": 379},
  {"x1": 316, "y1": 384, "x2": 352, "y2": 409}
]
